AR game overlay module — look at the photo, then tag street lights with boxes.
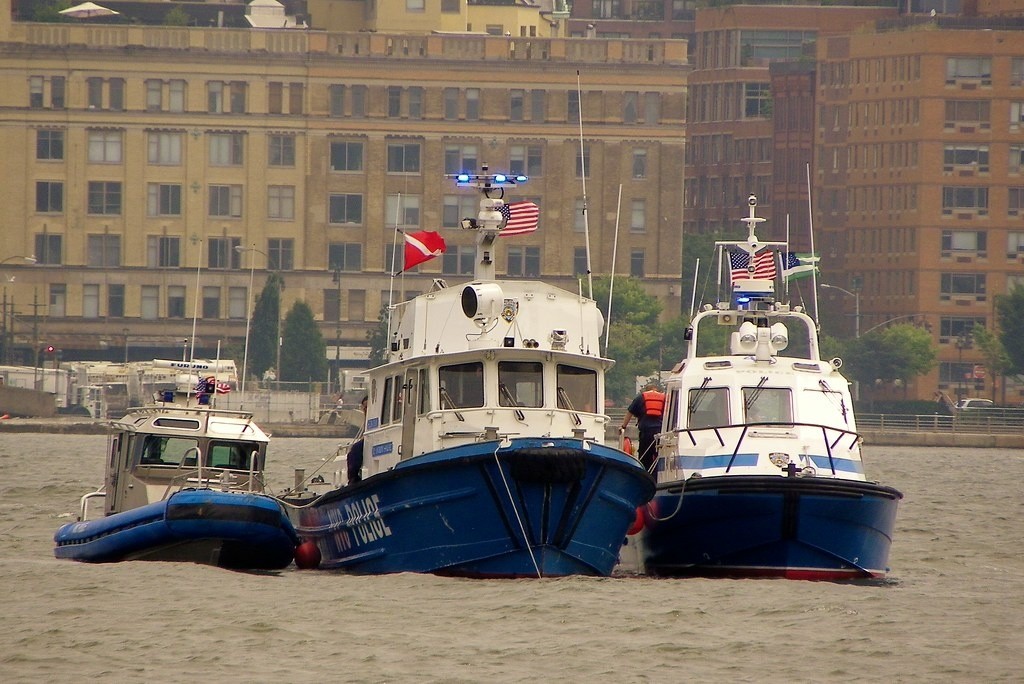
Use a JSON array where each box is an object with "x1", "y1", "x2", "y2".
[
  {"x1": 122, "y1": 327, "x2": 130, "y2": 364},
  {"x1": 954, "y1": 332, "x2": 977, "y2": 409},
  {"x1": 235, "y1": 245, "x2": 291, "y2": 385},
  {"x1": 333, "y1": 263, "x2": 342, "y2": 381},
  {"x1": 819, "y1": 282, "x2": 861, "y2": 401}
]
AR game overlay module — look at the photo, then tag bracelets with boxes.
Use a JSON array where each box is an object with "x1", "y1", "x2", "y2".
[{"x1": 622, "y1": 425, "x2": 626, "y2": 429}]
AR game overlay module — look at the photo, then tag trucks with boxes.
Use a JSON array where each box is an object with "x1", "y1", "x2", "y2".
[{"x1": 1, "y1": 348, "x2": 242, "y2": 422}]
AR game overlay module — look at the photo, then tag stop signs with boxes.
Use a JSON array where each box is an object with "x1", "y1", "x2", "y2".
[{"x1": 974, "y1": 365, "x2": 987, "y2": 379}]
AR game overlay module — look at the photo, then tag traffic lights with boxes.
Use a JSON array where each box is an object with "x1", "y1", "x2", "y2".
[{"x1": 48, "y1": 345, "x2": 55, "y2": 352}]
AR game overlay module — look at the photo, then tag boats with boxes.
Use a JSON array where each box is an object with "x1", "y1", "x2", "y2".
[
  {"x1": 643, "y1": 152, "x2": 909, "y2": 585},
  {"x1": 55, "y1": 242, "x2": 301, "y2": 574},
  {"x1": 280, "y1": 66, "x2": 657, "y2": 582}
]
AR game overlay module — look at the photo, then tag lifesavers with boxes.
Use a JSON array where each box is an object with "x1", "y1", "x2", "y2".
[{"x1": 623, "y1": 438, "x2": 632, "y2": 455}]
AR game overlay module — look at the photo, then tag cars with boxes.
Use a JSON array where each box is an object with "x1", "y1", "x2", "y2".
[{"x1": 954, "y1": 398, "x2": 994, "y2": 409}]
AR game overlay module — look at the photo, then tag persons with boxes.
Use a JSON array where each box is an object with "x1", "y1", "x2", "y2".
[
  {"x1": 337, "y1": 395, "x2": 344, "y2": 409},
  {"x1": 619, "y1": 384, "x2": 666, "y2": 471},
  {"x1": 932, "y1": 390, "x2": 944, "y2": 403}
]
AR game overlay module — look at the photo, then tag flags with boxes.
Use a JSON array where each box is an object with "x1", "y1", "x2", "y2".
[
  {"x1": 729, "y1": 251, "x2": 777, "y2": 287},
  {"x1": 781, "y1": 252, "x2": 821, "y2": 283},
  {"x1": 404, "y1": 230, "x2": 447, "y2": 271},
  {"x1": 197, "y1": 377, "x2": 231, "y2": 398},
  {"x1": 495, "y1": 201, "x2": 540, "y2": 236}
]
